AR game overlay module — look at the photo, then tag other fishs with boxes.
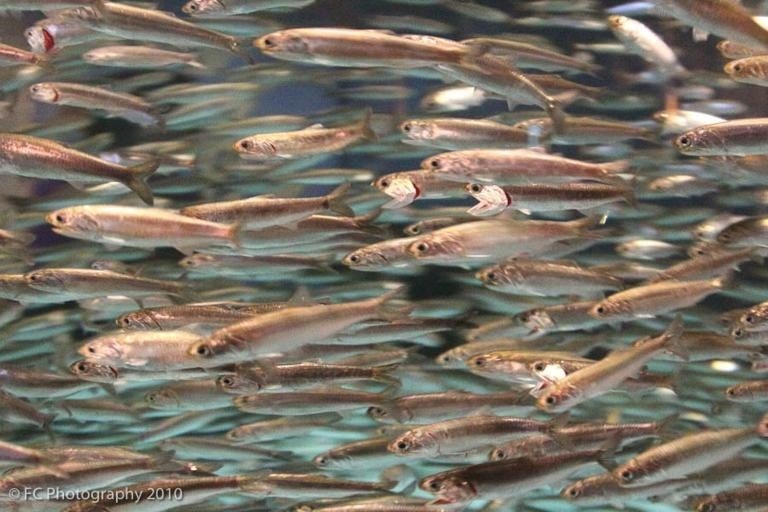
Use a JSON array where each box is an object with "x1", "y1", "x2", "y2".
[{"x1": 0, "y1": 0, "x2": 768, "y2": 512}]
